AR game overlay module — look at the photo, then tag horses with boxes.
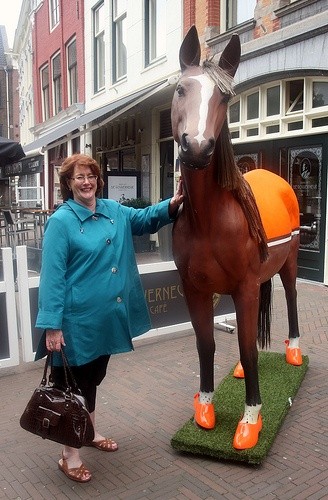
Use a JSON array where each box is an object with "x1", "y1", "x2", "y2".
[{"x1": 170, "y1": 25, "x2": 304, "y2": 450}]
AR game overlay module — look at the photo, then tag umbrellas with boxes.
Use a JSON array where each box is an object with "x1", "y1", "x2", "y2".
[{"x1": 0, "y1": 136, "x2": 26, "y2": 167}]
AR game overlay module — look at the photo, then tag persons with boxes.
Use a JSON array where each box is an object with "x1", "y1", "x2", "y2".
[
  {"x1": 118, "y1": 193, "x2": 126, "y2": 203},
  {"x1": 35, "y1": 153, "x2": 183, "y2": 482}
]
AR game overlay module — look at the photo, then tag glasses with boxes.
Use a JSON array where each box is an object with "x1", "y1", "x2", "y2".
[{"x1": 69, "y1": 175, "x2": 98, "y2": 182}]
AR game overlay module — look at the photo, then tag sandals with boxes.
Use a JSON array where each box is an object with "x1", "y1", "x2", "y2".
[
  {"x1": 57, "y1": 450, "x2": 92, "y2": 483},
  {"x1": 90, "y1": 436, "x2": 118, "y2": 451}
]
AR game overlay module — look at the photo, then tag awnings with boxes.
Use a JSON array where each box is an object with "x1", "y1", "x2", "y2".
[{"x1": 18, "y1": 79, "x2": 168, "y2": 159}]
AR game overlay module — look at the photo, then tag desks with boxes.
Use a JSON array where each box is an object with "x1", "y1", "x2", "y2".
[{"x1": 23, "y1": 209, "x2": 55, "y2": 248}]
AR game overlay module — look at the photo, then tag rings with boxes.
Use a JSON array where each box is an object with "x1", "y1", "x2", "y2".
[
  {"x1": 49, "y1": 342, "x2": 52, "y2": 346},
  {"x1": 174, "y1": 200, "x2": 177, "y2": 206},
  {"x1": 55, "y1": 341, "x2": 60, "y2": 344}
]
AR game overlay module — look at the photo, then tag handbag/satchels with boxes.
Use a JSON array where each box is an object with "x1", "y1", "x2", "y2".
[{"x1": 19, "y1": 346, "x2": 95, "y2": 449}]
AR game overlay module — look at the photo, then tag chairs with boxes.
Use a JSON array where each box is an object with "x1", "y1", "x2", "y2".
[{"x1": 0, "y1": 205, "x2": 58, "y2": 250}]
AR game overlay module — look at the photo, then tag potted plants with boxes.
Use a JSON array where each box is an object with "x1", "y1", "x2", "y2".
[{"x1": 121, "y1": 198, "x2": 152, "y2": 253}]
